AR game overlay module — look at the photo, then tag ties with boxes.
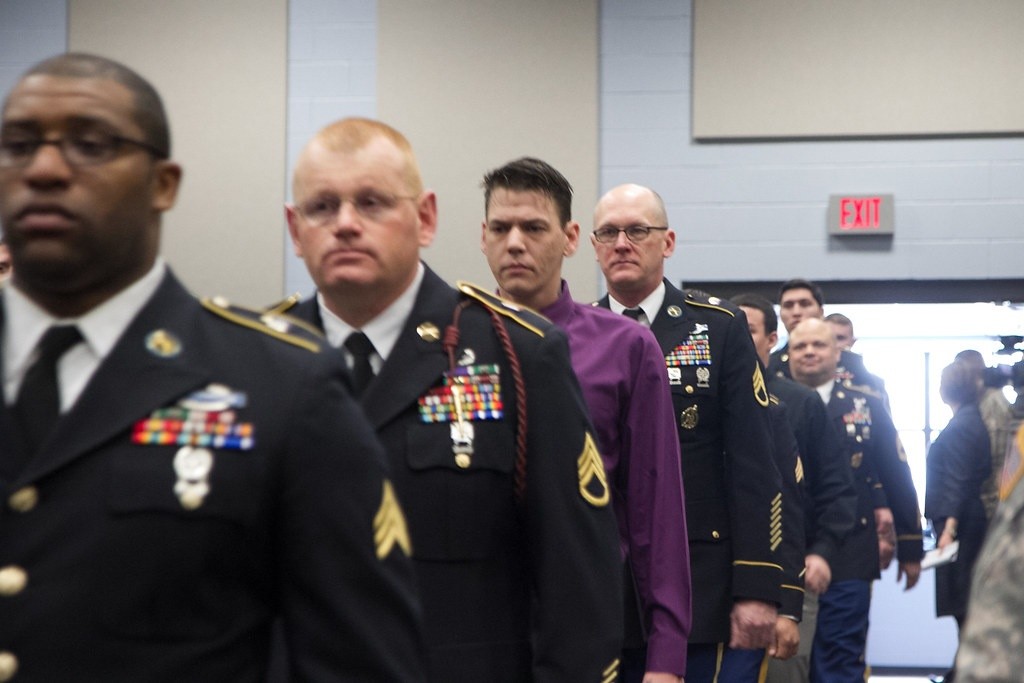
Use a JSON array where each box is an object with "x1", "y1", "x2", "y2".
[
  {"x1": 345, "y1": 332, "x2": 377, "y2": 397},
  {"x1": 623, "y1": 307, "x2": 644, "y2": 321},
  {"x1": 10, "y1": 324, "x2": 83, "y2": 447}
]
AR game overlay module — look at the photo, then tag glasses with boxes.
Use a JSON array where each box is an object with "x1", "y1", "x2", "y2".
[
  {"x1": 0, "y1": 127, "x2": 168, "y2": 168},
  {"x1": 593, "y1": 225, "x2": 668, "y2": 243}
]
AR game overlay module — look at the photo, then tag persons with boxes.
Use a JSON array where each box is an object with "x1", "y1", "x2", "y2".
[
  {"x1": 726, "y1": 277, "x2": 924, "y2": 683},
  {"x1": 926, "y1": 350, "x2": 1023, "y2": 683},
  {"x1": 584, "y1": 182, "x2": 787, "y2": 683},
  {"x1": 479, "y1": 154, "x2": 691, "y2": 683},
  {"x1": 0, "y1": 51, "x2": 438, "y2": 683},
  {"x1": 265, "y1": 113, "x2": 629, "y2": 683},
  {"x1": 0, "y1": 239, "x2": 14, "y2": 288}
]
